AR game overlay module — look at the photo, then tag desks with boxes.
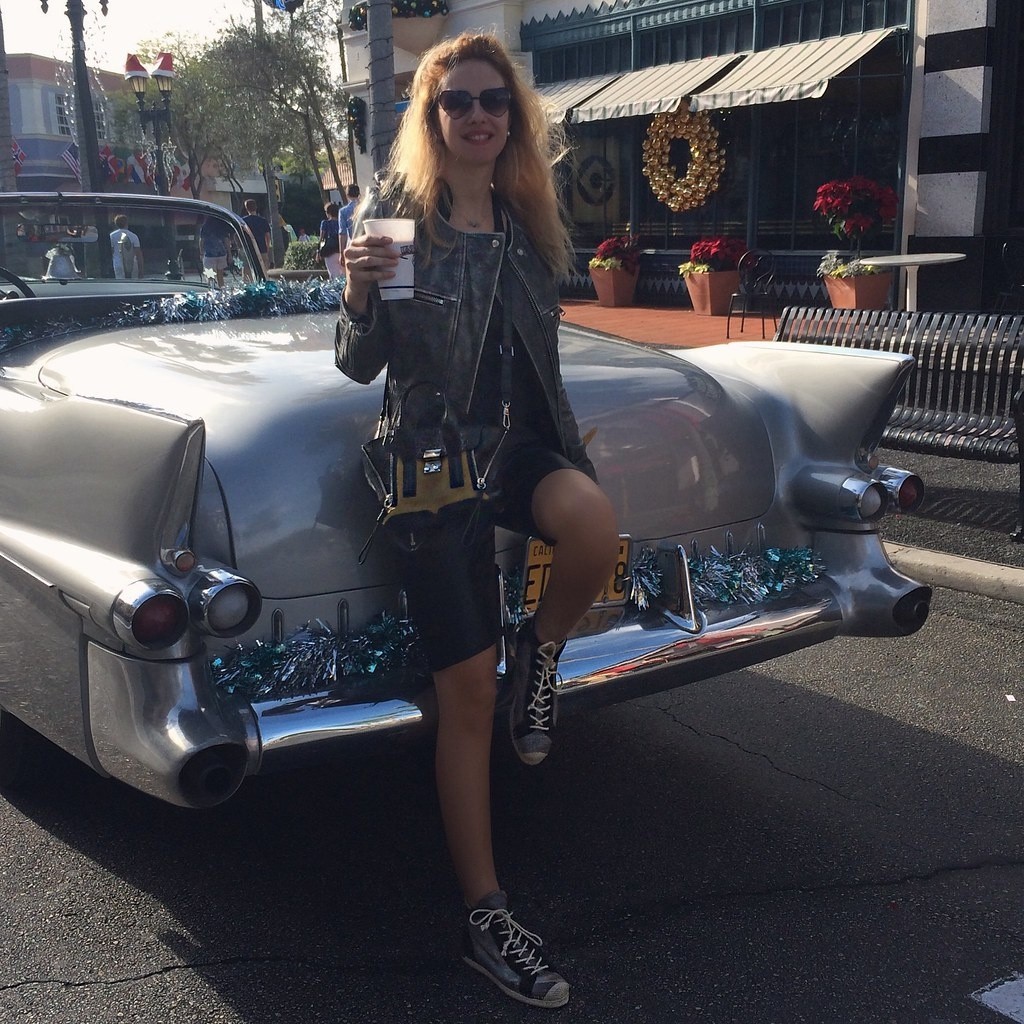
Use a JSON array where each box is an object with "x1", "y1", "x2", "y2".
[{"x1": 860, "y1": 253, "x2": 968, "y2": 344}]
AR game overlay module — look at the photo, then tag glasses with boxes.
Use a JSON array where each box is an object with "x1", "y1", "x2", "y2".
[{"x1": 435, "y1": 86, "x2": 512, "y2": 119}]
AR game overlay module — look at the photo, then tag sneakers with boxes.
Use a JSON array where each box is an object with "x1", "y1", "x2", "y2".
[
  {"x1": 457, "y1": 887, "x2": 570, "y2": 1010},
  {"x1": 509, "y1": 615, "x2": 568, "y2": 766}
]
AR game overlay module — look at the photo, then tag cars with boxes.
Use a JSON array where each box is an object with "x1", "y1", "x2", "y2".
[{"x1": 0, "y1": 188, "x2": 936, "y2": 820}]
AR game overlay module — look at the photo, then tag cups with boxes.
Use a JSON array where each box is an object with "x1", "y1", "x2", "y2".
[{"x1": 363, "y1": 218, "x2": 416, "y2": 300}]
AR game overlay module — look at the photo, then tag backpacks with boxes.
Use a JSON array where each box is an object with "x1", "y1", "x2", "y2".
[{"x1": 49, "y1": 248, "x2": 75, "y2": 277}]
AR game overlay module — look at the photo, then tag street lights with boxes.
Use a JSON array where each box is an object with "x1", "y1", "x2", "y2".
[
  {"x1": 38, "y1": 0, "x2": 111, "y2": 278},
  {"x1": 129, "y1": 75, "x2": 184, "y2": 280}
]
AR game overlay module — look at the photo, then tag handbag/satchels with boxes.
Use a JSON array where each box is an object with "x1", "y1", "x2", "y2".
[
  {"x1": 359, "y1": 379, "x2": 503, "y2": 534},
  {"x1": 319, "y1": 220, "x2": 338, "y2": 257}
]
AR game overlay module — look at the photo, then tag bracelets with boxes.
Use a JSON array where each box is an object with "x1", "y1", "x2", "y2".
[
  {"x1": 317, "y1": 249, "x2": 320, "y2": 252},
  {"x1": 200, "y1": 254, "x2": 204, "y2": 255}
]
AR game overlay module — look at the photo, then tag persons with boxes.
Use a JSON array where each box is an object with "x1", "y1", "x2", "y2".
[
  {"x1": 11, "y1": 184, "x2": 359, "y2": 290},
  {"x1": 332, "y1": 33, "x2": 617, "y2": 1010}
]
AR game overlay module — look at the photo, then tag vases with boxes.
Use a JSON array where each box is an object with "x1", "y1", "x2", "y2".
[
  {"x1": 825, "y1": 271, "x2": 893, "y2": 311},
  {"x1": 684, "y1": 271, "x2": 740, "y2": 315},
  {"x1": 589, "y1": 267, "x2": 640, "y2": 307}
]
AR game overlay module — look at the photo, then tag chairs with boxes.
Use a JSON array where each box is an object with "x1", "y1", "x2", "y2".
[
  {"x1": 726, "y1": 247, "x2": 777, "y2": 338},
  {"x1": 994, "y1": 242, "x2": 1024, "y2": 315}
]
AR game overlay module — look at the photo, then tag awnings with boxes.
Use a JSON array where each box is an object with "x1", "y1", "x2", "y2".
[{"x1": 527, "y1": 29, "x2": 898, "y2": 123}]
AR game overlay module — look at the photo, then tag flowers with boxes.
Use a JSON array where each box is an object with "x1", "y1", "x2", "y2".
[
  {"x1": 588, "y1": 235, "x2": 641, "y2": 277},
  {"x1": 812, "y1": 174, "x2": 900, "y2": 279},
  {"x1": 676, "y1": 235, "x2": 751, "y2": 278}
]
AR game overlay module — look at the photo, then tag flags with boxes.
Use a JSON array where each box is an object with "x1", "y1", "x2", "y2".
[{"x1": 10, "y1": 136, "x2": 190, "y2": 190}]
